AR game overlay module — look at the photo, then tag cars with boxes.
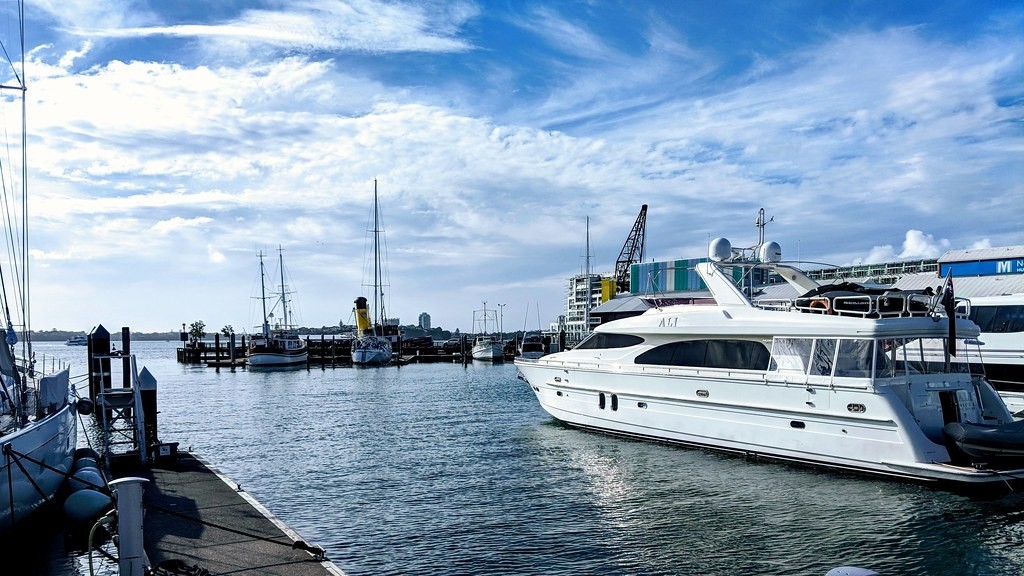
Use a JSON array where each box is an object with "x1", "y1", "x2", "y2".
[{"x1": 443, "y1": 338, "x2": 461, "y2": 349}]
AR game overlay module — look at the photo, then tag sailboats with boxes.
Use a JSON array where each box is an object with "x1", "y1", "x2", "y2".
[
  {"x1": 471, "y1": 302, "x2": 505, "y2": 363},
  {"x1": 244, "y1": 242, "x2": 308, "y2": 367},
  {"x1": 350, "y1": 177, "x2": 394, "y2": 366},
  {"x1": 0, "y1": 0, "x2": 95, "y2": 535}
]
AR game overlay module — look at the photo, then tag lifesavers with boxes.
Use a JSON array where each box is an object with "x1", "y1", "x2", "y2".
[{"x1": 809, "y1": 300, "x2": 832, "y2": 314}]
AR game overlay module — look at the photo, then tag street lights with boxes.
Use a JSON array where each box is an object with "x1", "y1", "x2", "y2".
[{"x1": 497, "y1": 303, "x2": 506, "y2": 343}]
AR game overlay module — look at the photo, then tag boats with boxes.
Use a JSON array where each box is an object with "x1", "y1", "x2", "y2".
[
  {"x1": 65, "y1": 335, "x2": 88, "y2": 347},
  {"x1": 512, "y1": 208, "x2": 1024, "y2": 497},
  {"x1": 521, "y1": 340, "x2": 546, "y2": 359}
]
